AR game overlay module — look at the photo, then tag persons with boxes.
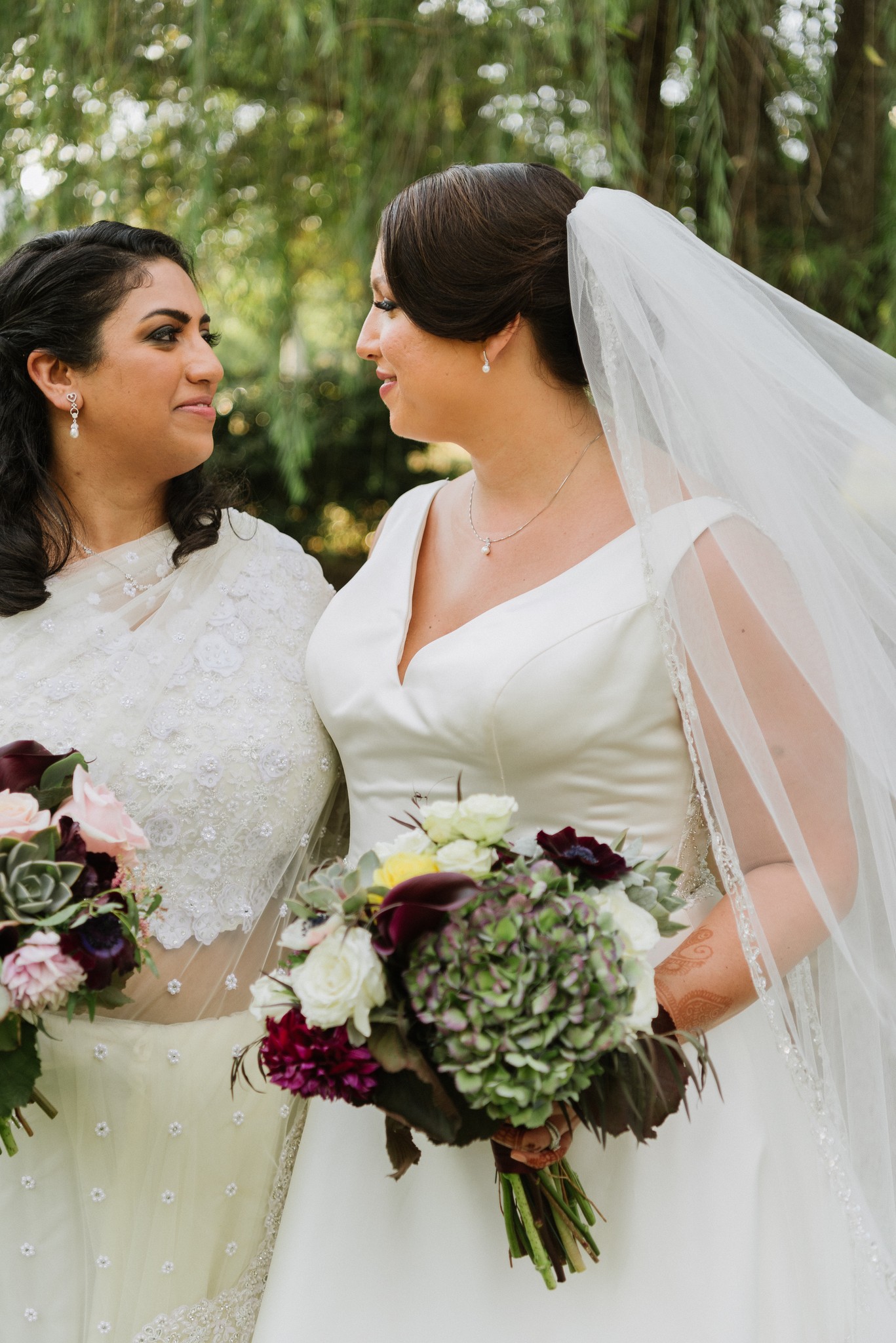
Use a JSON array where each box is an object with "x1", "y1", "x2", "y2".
[
  {"x1": 0, "y1": 222, "x2": 337, "y2": 1343},
  {"x1": 303, "y1": 157, "x2": 893, "y2": 1343}
]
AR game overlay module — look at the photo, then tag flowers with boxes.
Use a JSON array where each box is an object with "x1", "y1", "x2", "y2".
[
  {"x1": 231, "y1": 761, "x2": 730, "y2": 1295},
  {"x1": 0, "y1": 735, "x2": 179, "y2": 1153}
]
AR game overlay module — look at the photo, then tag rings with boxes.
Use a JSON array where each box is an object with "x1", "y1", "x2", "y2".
[{"x1": 545, "y1": 1120, "x2": 562, "y2": 1152}]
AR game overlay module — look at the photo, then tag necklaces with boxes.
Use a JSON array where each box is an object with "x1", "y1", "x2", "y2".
[
  {"x1": 469, "y1": 431, "x2": 604, "y2": 554},
  {"x1": 36, "y1": 486, "x2": 178, "y2": 590}
]
